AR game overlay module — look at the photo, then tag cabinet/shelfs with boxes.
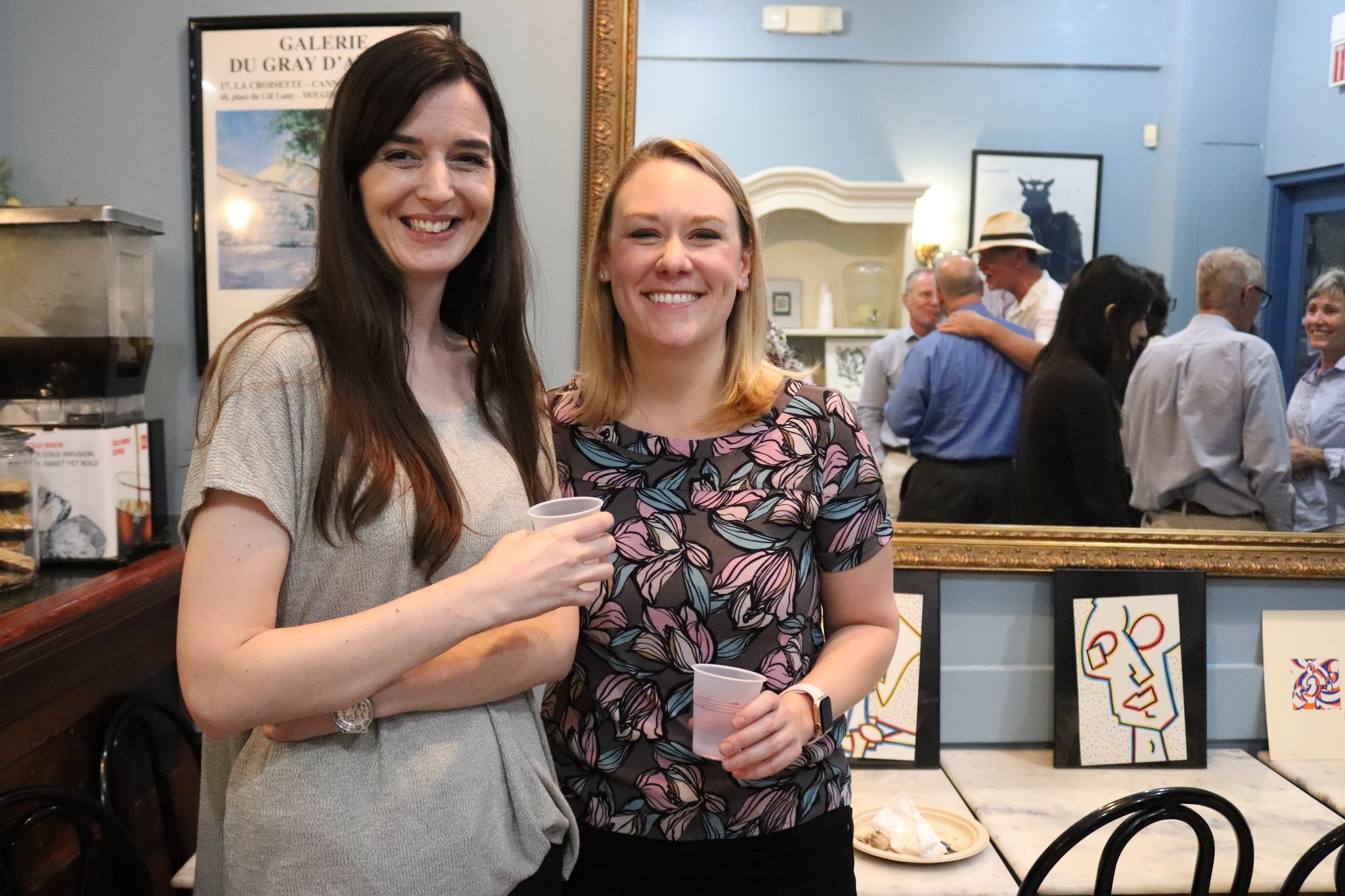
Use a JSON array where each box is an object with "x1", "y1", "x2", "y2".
[{"x1": 742, "y1": 165, "x2": 931, "y2": 412}]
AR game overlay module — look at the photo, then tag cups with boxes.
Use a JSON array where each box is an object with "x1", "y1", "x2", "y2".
[
  {"x1": 526, "y1": 495, "x2": 604, "y2": 591},
  {"x1": 691, "y1": 663, "x2": 768, "y2": 761}
]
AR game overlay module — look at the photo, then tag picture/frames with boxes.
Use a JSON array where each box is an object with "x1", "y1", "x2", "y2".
[
  {"x1": 823, "y1": 337, "x2": 875, "y2": 404},
  {"x1": 185, "y1": 11, "x2": 461, "y2": 383},
  {"x1": 1052, "y1": 563, "x2": 1206, "y2": 772},
  {"x1": 837, "y1": 568, "x2": 942, "y2": 768},
  {"x1": 766, "y1": 279, "x2": 804, "y2": 327},
  {"x1": 969, "y1": 149, "x2": 1102, "y2": 285}
]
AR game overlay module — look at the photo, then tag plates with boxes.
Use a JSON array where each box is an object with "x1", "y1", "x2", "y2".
[{"x1": 852, "y1": 805, "x2": 990, "y2": 864}]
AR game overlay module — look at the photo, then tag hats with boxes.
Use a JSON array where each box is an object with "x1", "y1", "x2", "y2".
[{"x1": 968, "y1": 211, "x2": 1052, "y2": 256}]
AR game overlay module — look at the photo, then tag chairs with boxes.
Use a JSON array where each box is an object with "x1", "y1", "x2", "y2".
[
  {"x1": 1014, "y1": 785, "x2": 1255, "y2": 896},
  {"x1": 0, "y1": 694, "x2": 198, "y2": 896}
]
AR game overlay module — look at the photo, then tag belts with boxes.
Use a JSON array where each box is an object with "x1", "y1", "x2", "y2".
[
  {"x1": 915, "y1": 452, "x2": 1016, "y2": 467},
  {"x1": 1166, "y1": 498, "x2": 1261, "y2": 518}
]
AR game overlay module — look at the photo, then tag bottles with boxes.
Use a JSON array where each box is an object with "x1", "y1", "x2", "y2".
[
  {"x1": 0, "y1": 424, "x2": 41, "y2": 594},
  {"x1": 815, "y1": 283, "x2": 834, "y2": 330}
]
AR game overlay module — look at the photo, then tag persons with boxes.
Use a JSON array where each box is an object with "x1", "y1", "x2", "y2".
[
  {"x1": 544, "y1": 137, "x2": 899, "y2": 896},
  {"x1": 1119, "y1": 247, "x2": 1298, "y2": 532},
  {"x1": 1284, "y1": 272, "x2": 1345, "y2": 535},
  {"x1": 175, "y1": 24, "x2": 615, "y2": 896},
  {"x1": 863, "y1": 209, "x2": 1171, "y2": 526}
]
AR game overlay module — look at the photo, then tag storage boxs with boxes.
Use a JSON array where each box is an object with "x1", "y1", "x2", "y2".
[{"x1": 28, "y1": 421, "x2": 156, "y2": 560}]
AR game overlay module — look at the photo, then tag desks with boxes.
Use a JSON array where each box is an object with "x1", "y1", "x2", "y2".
[{"x1": 849, "y1": 739, "x2": 1345, "y2": 896}]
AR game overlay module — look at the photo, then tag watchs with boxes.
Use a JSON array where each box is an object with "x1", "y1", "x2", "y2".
[
  {"x1": 332, "y1": 698, "x2": 373, "y2": 733},
  {"x1": 778, "y1": 683, "x2": 833, "y2": 746}
]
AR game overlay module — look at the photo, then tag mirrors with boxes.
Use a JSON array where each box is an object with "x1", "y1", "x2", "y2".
[{"x1": 578, "y1": 0, "x2": 1345, "y2": 572}]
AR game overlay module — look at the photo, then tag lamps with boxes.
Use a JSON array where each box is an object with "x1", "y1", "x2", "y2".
[{"x1": 915, "y1": 245, "x2": 941, "y2": 265}]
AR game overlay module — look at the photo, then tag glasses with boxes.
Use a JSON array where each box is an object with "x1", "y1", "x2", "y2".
[
  {"x1": 1167, "y1": 298, "x2": 1177, "y2": 311},
  {"x1": 1241, "y1": 286, "x2": 1274, "y2": 309}
]
